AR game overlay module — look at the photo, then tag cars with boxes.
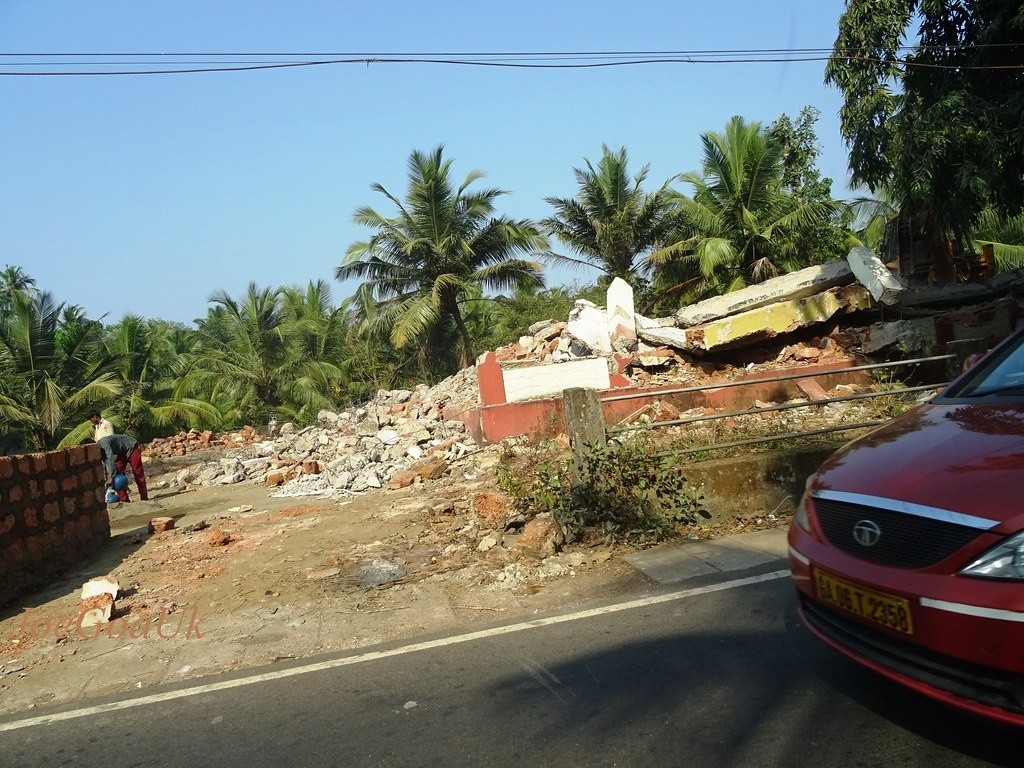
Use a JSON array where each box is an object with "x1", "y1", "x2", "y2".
[{"x1": 786, "y1": 326, "x2": 1024, "y2": 729}]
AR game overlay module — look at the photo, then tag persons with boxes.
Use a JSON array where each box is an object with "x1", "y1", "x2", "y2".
[
  {"x1": 80, "y1": 434, "x2": 149, "y2": 503},
  {"x1": 86, "y1": 409, "x2": 113, "y2": 443}
]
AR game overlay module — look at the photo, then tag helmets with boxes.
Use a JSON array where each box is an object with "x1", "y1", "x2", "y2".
[
  {"x1": 114, "y1": 474, "x2": 128, "y2": 490},
  {"x1": 108, "y1": 495, "x2": 120, "y2": 502}
]
